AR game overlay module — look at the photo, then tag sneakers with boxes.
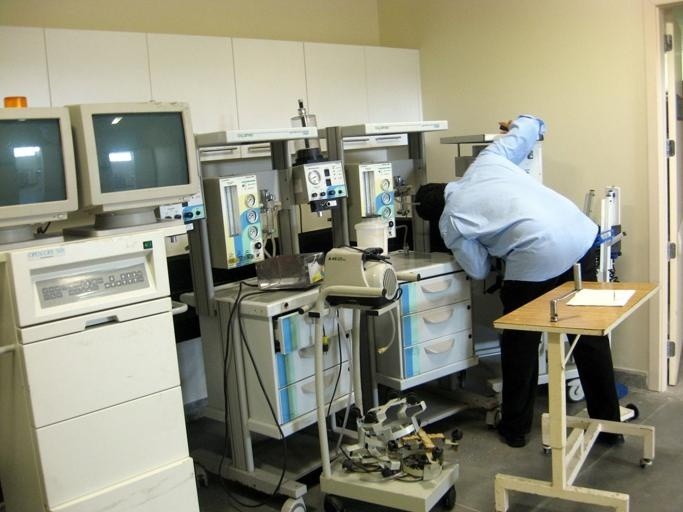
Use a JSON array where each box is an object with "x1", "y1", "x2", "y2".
[
  {"x1": 498, "y1": 419, "x2": 526, "y2": 447},
  {"x1": 595, "y1": 432, "x2": 626, "y2": 446}
]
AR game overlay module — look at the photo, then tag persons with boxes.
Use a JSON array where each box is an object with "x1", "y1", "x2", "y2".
[{"x1": 414, "y1": 114, "x2": 626, "y2": 449}]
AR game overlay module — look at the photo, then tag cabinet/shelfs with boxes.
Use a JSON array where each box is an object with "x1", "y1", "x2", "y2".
[
  {"x1": 240, "y1": 303, "x2": 355, "y2": 439},
  {"x1": 1, "y1": 30, "x2": 150, "y2": 108},
  {"x1": 146, "y1": 33, "x2": 309, "y2": 163},
  {"x1": 375, "y1": 271, "x2": 480, "y2": 390},
  {"x1": 304, "y1": 41, "x2": 423, "y2": 152}
]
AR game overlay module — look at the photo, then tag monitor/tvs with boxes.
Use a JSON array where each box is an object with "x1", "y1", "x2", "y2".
[
  {"x1": 0, "y1": 107, "x2": 78, "y2": 252},
  {"x1": 66, "y1": 101, "x2": 198, "y2": 236}
]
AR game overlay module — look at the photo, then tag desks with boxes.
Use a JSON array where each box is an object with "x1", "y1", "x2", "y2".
[{"x1": 494, "y1": 281, "x2": 661, "y2": 512}]
